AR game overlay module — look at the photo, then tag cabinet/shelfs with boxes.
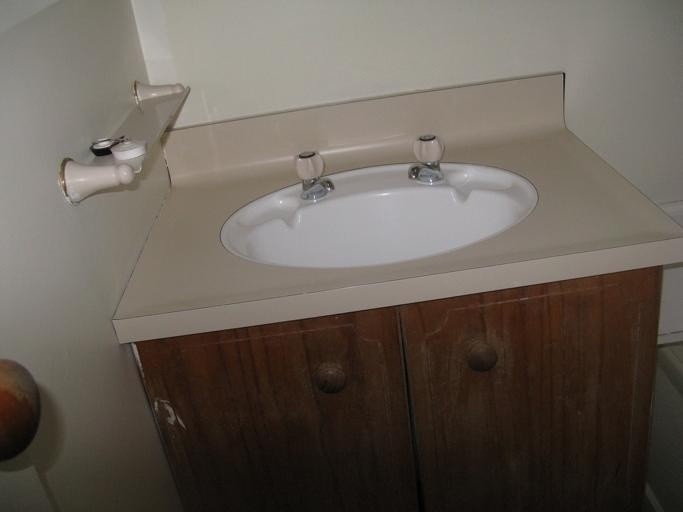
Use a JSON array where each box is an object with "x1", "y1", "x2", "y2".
[{"x1": 129, "y1": 267, "x2": 663, "y2": 509}]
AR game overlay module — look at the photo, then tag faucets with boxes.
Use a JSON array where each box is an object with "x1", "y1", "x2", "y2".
[
  {"x1": 409, "y1": 135, "x2": 445, "y2": 185},
  {"x1": 296, "y1": 151, "x2": 336, "y2": 202}
]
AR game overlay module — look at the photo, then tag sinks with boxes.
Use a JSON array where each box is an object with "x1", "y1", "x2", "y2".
[{"x1": 218, "y1": 158, "x2": 539, "y2": 272}]
